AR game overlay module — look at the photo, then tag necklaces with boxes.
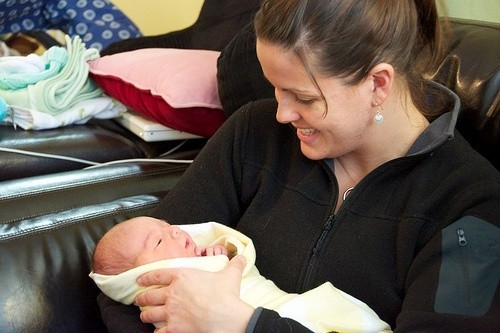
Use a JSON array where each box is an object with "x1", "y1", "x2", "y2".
[{"x1": 342, "y1": 186, "x2": 354, "y2": 200}]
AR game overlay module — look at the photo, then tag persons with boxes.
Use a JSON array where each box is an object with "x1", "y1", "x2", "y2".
[
  {"x1": 97, "y1": 0, "x2": 500, "y2": 333},
  {"x1": 89, "y1": 217, "x2": 392, "y2": 333}
]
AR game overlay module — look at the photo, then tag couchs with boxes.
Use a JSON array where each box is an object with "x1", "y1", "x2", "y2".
[
  {"x1": 0, "y1": 0, "x2": 144, "y2": 54},
  {"x1": 100, "y1": 0, "x2": 266, "y2": 57},
  {"x1": 215, "y1": 13, "x2": 500, "y2": 169},
  {"x1": 0, "y1": 119, "x2": 212, "y2": 333}
]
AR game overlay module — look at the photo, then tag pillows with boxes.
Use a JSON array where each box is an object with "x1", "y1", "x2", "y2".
[{"x1": 87, "y1": 48, "x2": 225, "y2": 136}]
